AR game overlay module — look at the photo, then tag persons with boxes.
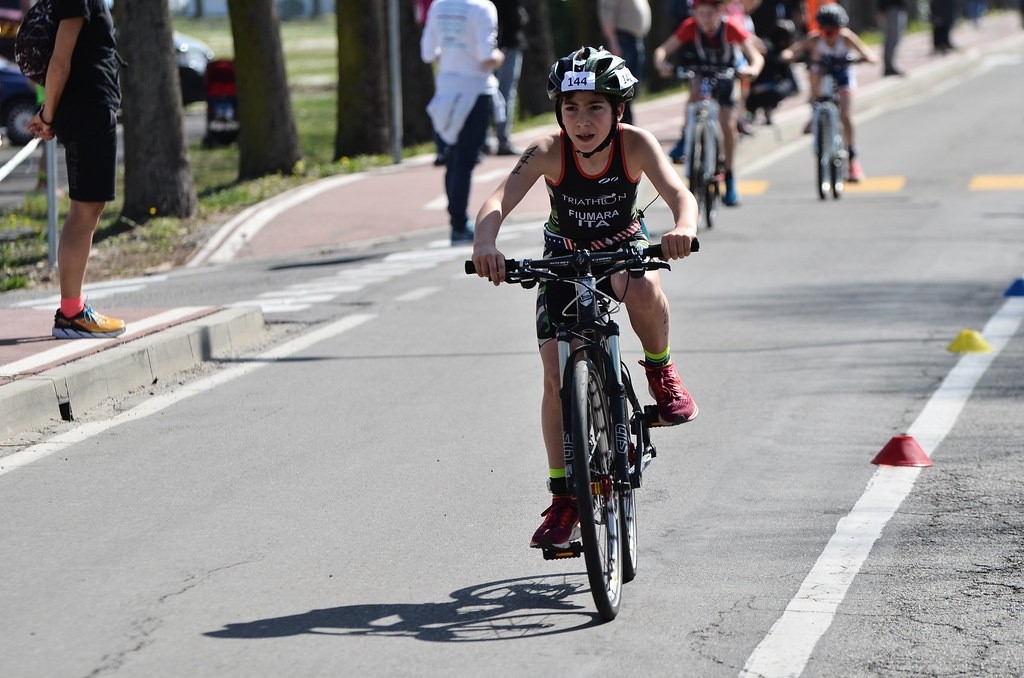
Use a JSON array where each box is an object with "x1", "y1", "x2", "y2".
[
  {"x1": 657, "y1": 0, "x2": 877, "y2": 209},
  {"x1": 471, "y1": 47, "x2": 697, "y2": 551},
  {"x1": 875, "y1": 0, "x2": 957, "y2": 75},
  {"x1": 600, "y1": 0, "x2": 652, "y2": 125},
  {"x1": 416, "y1": 0, "x2": 529, "y2": 244},
  {"x1": 27, "y1": 0, "x2": 126, "y2": 338}
]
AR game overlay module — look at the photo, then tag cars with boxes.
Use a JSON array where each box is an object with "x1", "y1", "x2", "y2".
[
  {"x1": 0, "y1": 53, "x2": 45, "y2": 146},
  {"x1": 0, "y1": 0, "x2": 214, "y2": 104}
]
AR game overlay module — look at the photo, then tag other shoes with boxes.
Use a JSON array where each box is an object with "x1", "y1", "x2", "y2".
[
  {"x1": 497, "y1": 143, "x2": 519, "y2": 154},
  {"x1": 803, "y1": 120, "x2": 813, "y2": 135},
  {"x1": 668, "y1": 140, "x2": 686, "y2": 160},
  {"x1": 449, "y1": 227, "x2": 474, "y2": 245},
  {"x1": 722, "y1": 178, "x2": 739, "y2": 207},
  {"x1": 435, "y1": 151, "x2": 446, "y2": 165},
  {"x1": 845, "y1": 158, "x2": 861, "y2": 182}
]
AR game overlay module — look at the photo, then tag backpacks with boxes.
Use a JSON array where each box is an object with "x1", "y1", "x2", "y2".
[{"x1": 15, "y1": 0, "x2": 59, "y2": 89}]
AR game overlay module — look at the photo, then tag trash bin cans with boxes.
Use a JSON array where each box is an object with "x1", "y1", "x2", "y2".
[{"x1": 202, "y1": 59, "x2": 240, "y2": 151}]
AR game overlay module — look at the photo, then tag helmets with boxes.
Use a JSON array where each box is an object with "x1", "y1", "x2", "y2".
[
  {"x1": 546, "y1": 47, "x2": 639, "y2": 105},
  {"x1": 814, "y1": 4, "x2": 850, "y2": 28},
  {"x1": 687, "y1": 0, "x2": 725, "y2": 11}
]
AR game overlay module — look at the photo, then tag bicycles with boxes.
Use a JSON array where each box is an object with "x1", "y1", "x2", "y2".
[
  {"x1": 782, "y1": 58, "x2": 873, "y2": 201},
  {"x1": 464, "y1": 237, "x2": 703, "y2": 618},
  {"x1": 670, "y1": 63, "x2": 754, "y2": 229}
]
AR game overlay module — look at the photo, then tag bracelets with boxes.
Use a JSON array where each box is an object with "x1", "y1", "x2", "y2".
[{"x1": 39, "y1": 108, "x2": 55, "y2": 125}]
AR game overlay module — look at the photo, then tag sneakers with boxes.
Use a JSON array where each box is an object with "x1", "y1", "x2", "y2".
[
  {"x1": 52, "y1": 304, "x2": 125, "y2": 339},
  {"x1": 530, "y1": 492, "x2": 579, "y2": 548},
  {"x1": 637, "y1": 358, "x2": 698, "y2": 427}
]
